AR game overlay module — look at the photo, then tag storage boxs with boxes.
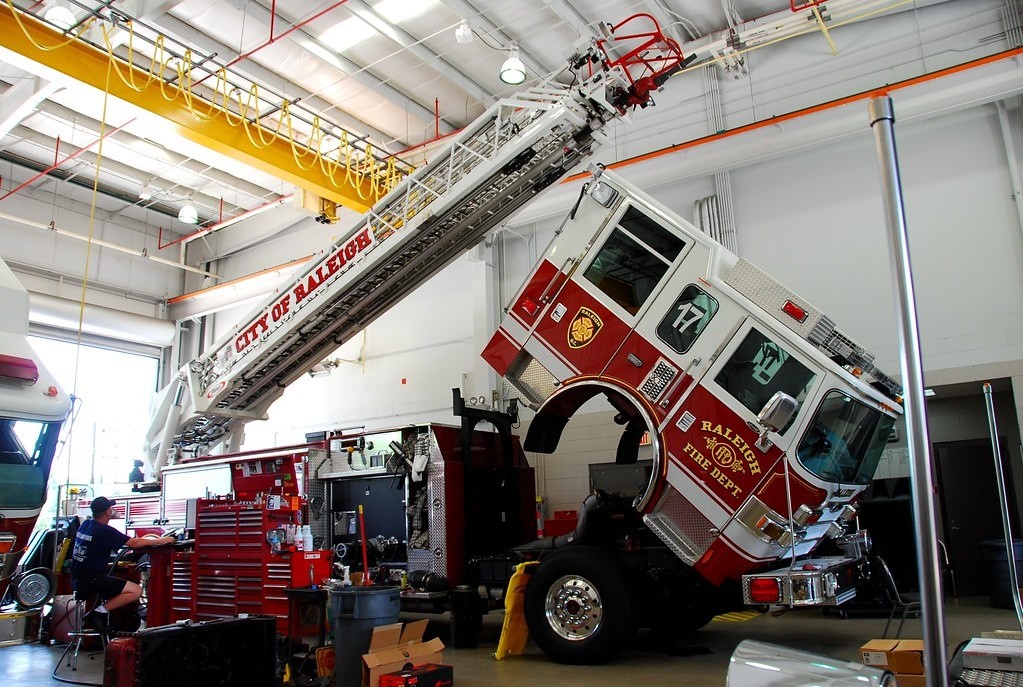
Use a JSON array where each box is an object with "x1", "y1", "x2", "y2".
[
  {"x1": 380, "y1": 663, "x2": 454, "y2": 687},
  {"x1": 859, "y1": 639, "x2": 927, "y2": 687}
]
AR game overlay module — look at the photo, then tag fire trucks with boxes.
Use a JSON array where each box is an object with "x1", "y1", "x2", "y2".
[
  {"x1": 51, "y1": 11, "x2": 936, "y2": 666},
  {"x1": 0, "y1": 253, "x2": 82, "y2": 616}
]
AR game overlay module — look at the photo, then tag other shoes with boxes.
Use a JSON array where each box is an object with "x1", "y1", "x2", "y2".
[{"x1": 83, "y1": 610, "x2": 112, "y2": 633}]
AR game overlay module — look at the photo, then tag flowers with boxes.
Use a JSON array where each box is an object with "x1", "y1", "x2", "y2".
[{"x1": 67, "y1": 488, "x2": 80, "y2": 494}]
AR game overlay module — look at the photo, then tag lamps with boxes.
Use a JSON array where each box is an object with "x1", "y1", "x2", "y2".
[
  {"x1": 453, "y1": 22, "x2": 475, "y2": 44},
  {"x1": 137, "y1": 184, "x2": 152, "y2": 200},
  {"x1": 499, "y1": 40, "x2": 526, "y2": 86},
  {"x1": 178, "y1": 190, "x2": 199, "y2": 224},
  {"x1": 44, "y1": 6, "x2": 78, "y2": 39}
]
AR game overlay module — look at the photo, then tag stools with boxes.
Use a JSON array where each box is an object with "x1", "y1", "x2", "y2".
[{"x1": 67, "y1": 590, "x2": 111, "y2": 670}]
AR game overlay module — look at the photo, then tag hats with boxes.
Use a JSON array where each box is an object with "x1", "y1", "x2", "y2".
[{"x1": 91, "y1": 497, "x2": 116, "y2": 513}]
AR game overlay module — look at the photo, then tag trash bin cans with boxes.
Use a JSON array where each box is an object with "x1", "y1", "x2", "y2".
[
  {"x1": 329, "y1": 585, "x2": 401, "y2": 687},
  {"x1": 978, "y1": 539, "x2": 1023, "y2": 609}
]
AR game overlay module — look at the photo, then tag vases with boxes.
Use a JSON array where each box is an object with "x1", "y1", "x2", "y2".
[{"x1": 71, "y1": 494, "x2": 77, "y2": 501}]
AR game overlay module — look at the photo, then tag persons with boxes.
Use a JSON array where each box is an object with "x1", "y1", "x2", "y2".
[{"x1": 71, "y1": 497, "x2": 175, "y2": 633}]
